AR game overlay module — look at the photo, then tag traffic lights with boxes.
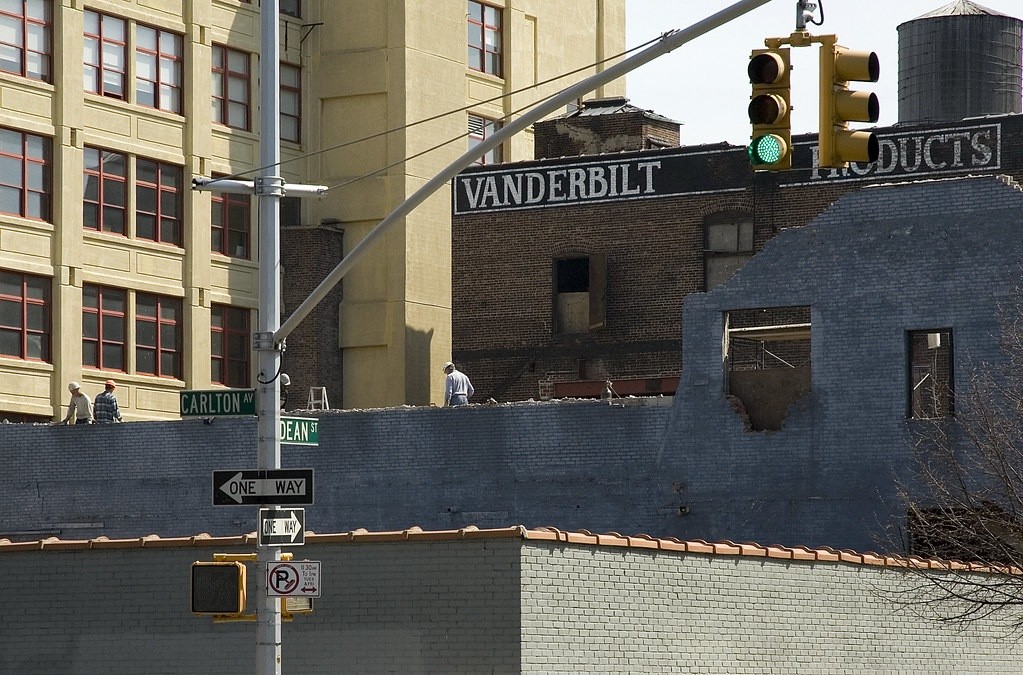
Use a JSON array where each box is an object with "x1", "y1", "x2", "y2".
[
  {"x1": 188, "y1": 561, "x2": 248, "y2": 617},
  {"x1": 748, "y1": 48, "x2": 793, "y2": 172},
  {"x1": 279, "y1": 598, "x2": 314, "y2": 617},
  {"x1": 816, "y1": 43, "x2": 880, "y2": 169}
]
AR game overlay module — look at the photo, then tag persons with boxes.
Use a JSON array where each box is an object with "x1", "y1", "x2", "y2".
[
  {"x1": 280, "y1": 374, "x2": 291, "y2": 411},
  {"x1": 93, "y1": 380, "x2": 124, "y2": 424},
  {"x1": 442, "y1": 362, "x2": 474, "y2": 406},
  {"x1": 62, "y1": 382, "x2": 100, "y2": 425}
]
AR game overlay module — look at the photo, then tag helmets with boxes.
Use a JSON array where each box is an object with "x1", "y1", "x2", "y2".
[
  {"x1": 69, "y1": 382, "x2": 81, "y2": 390},
  {"x1": 442, "y1": 362, "x2": 455, "y2": 373},
  {"x1": 104, "y1": 380, "x2": 116, "y2": 388}
]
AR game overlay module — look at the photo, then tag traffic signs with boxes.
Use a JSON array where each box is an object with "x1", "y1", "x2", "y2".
[
  {"x1": 211, "y1": 469, "x2": 316, "y2": 509},
  {"x1": 179, "y1": 389, "x2": 256, "y2": 418},
  {"x1": 278, "y1": 417, "x2": 321, "y2": 447},
  {"x1": 258, "y1": 509, "x2": 306, "y2": 546}
]
